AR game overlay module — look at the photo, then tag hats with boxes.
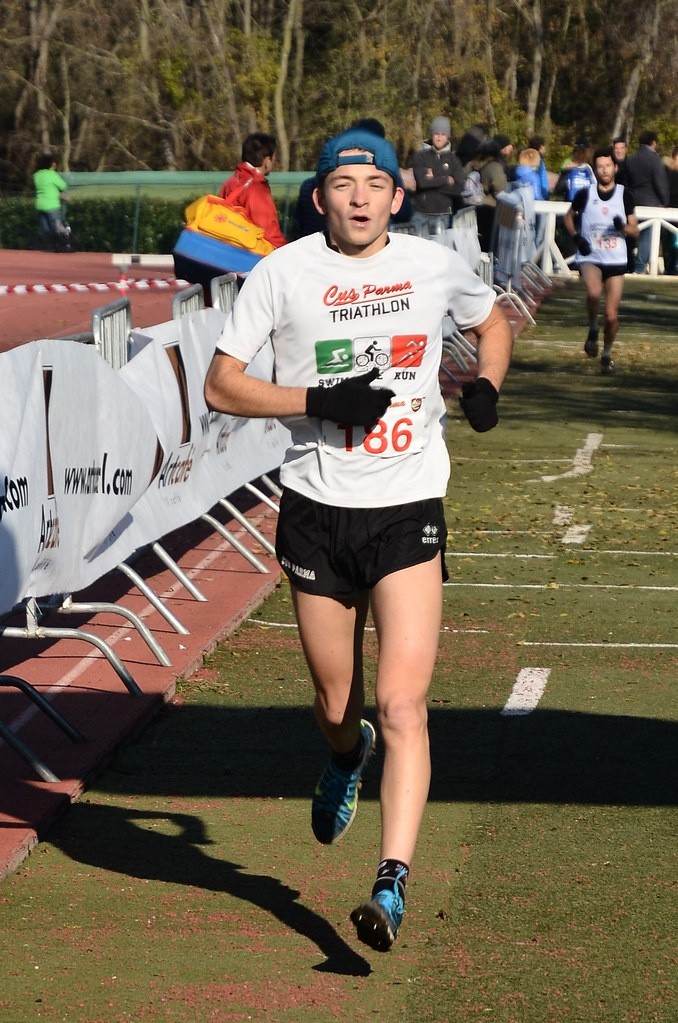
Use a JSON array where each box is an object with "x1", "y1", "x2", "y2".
[
  {"x1": 315, "y1": 128, "x2": 399, "y2": 189},
  {"x1": 493, "y1": 135, "x2": 510, "y2": 150},
  {"x1": 428, "y1": 116, "x2": 453, "y2": 137}
]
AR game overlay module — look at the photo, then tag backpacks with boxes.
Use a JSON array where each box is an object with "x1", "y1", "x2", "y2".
[
  {"x1": 460, "y1": 158, "x2": 499, "y2": 207},
  {"x1": 568, "y1": 167, "x2": 591, "y2": 201}
]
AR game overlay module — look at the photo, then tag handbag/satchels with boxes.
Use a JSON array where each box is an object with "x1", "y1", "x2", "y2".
[{"x1": 169, "y1": 192, "x2": 277, "y2": 290}]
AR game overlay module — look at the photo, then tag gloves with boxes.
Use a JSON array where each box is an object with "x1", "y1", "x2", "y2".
[
  {"x1": 614, "y1": 216, "x2": 625, "y2": 230},
  {"x1": 457, "y1": 377, "x2": 499, "y2": 433},
  {"x1": 304, "y1": 366, "x2": 396, "y2": 428},
  {"x1": 573, "y1": 233, "x2": 592, "y2": 256}
]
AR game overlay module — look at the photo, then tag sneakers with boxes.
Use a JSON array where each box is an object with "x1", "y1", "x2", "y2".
[
  {"x1": 352, "y1": 889, "x2": 403, "y2": 953},
  {"x1": 601, "y1": 355, "x2": 617, "y2": 373},
  {"x1": 585, "y1": 337, "x2": 599, "y2": 356},
  {"x1": 311, "y1": 718, "x2": 377, "y2": 846}
]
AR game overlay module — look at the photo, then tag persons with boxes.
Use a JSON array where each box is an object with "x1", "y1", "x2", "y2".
[
  {"x1": 290, "y1": 115, "x2": 678, "y2": 293},
  {"x1": 202, "y1": 132, "x2": 512, "y2": 953},
  {"x1": 565, "y1": 147, "x2": 640, "y2": 375},
  {"x1": 216, "y1": 133, "x2": 288, "y2": 248},
  {"x1": 30, "y1": 154, "x2": 73, "y2": 254}
]
